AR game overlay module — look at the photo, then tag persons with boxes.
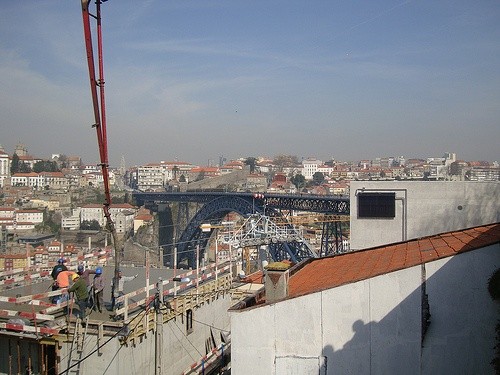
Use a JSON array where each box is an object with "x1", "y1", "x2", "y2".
[
  {"x1": 51, "y1": 258, "x2": 104, "y2": 322},
  {"x1": 111, "y1": 269, "x2": 139, "y2": 311}
]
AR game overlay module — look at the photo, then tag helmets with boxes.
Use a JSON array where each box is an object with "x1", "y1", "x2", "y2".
[
  {"x1": 56, "y1": 268, "x2": 61, "y2": 274},
  {"x1": 58, "y1": 259, "x2": 64, "y2": 263},
  {"x1": 95, "y1": 268, "x2": 102, "y2": 273},
  {"x1": 78, "y1": 266, "x2": 83, "y2": 270},
  {"x1": 71, "y1": 273, "x2": 79, "y2": 281}
]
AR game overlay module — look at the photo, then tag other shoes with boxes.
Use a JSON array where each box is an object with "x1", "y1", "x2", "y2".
[{"x1": 101, "y1": 310, "x2": 103, "y2": 314}]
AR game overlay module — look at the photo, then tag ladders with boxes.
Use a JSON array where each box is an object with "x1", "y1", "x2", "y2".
[{"x1": 67, "y1": 318, "x2": 89, "y2": 375}]
